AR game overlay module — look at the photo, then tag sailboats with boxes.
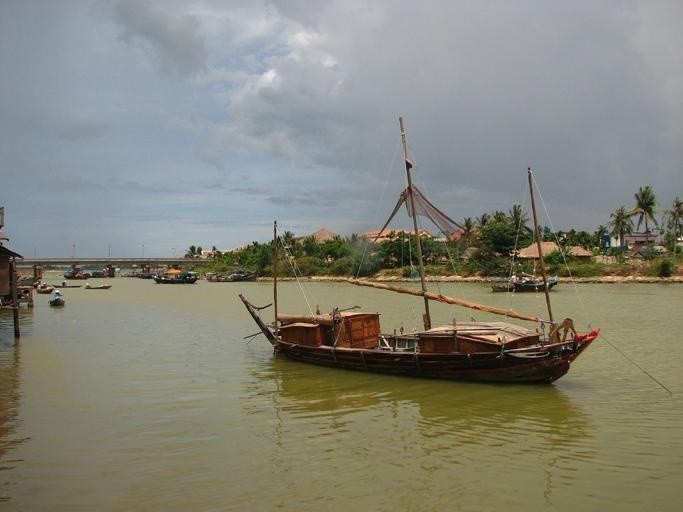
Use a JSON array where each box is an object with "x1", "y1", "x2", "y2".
[{"x1": 238, "y1": 116, "x2": 601, "y2": 385}]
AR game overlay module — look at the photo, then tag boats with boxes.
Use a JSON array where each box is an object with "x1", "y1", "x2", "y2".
[
  {"x1": 48, "y1": 296, "x2": 64, "y2": 306},
  {"x1": 32, "y1": 265, "x2": 257, "y2": 293},
  {"x1": 490, "y1": 275, "x2": 559, "y2": 292}
]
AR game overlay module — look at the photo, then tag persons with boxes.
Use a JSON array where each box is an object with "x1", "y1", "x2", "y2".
[{"x1": 54, "y1": 290, "x2": 62, "y2": 300}]
